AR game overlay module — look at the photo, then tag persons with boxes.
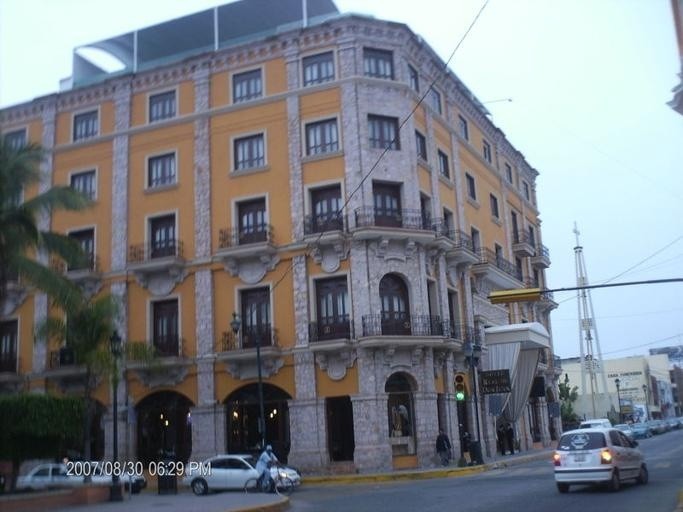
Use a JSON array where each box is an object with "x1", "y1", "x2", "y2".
[
  {"x1": 507, "y1": 424, "x2": 514, "y2": 455},
  {"x1": 497, "y1": 424, "x2": 506, "y2": 456},
  {"x1": 256, "y1": 445, "x2": 282, "y2": 493},
  {"x1": 436, "y1": 428, "x2": 452, "y2": 468}
]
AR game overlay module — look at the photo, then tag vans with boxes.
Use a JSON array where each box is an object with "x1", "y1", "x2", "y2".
[{"x1": 578, "y1": 418, "x2": 611, "y2": 430}]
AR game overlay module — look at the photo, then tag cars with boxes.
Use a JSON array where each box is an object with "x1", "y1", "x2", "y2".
[
  {"x1": 614, "y1": 416, "x2": 683, "y2": 445},
  {"x1": 553, "y1": 427, "x2": 649, "y2": 494},
  {"x1": 180, "y1": 452, "x2": 303, "y2": 497},
  {"x1": 6, "y1": 459, "x2": 145, "y2": 495}
]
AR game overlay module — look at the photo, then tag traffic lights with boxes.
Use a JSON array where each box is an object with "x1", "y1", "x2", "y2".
[{"x1": 454, "y1": 372, "x2": 464, "y2": 401}]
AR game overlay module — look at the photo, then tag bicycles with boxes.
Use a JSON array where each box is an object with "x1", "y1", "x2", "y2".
[{"x1": 238, "y1": 462, "x2": 294, "y2": 499}]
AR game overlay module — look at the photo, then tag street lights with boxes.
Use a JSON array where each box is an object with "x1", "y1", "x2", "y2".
[
  {"x1": 105, "y1": 329, "x2": 126, "y2": 504},
  {"x1": 640, "y1": 383, "x2": 652, "y2": 422},
  {"x1": 614, "y1": 378, "x2": 624, "y2": 425},
  {"x1": 228, "y1": 310, "x2": 271, "y2": 459}
]
